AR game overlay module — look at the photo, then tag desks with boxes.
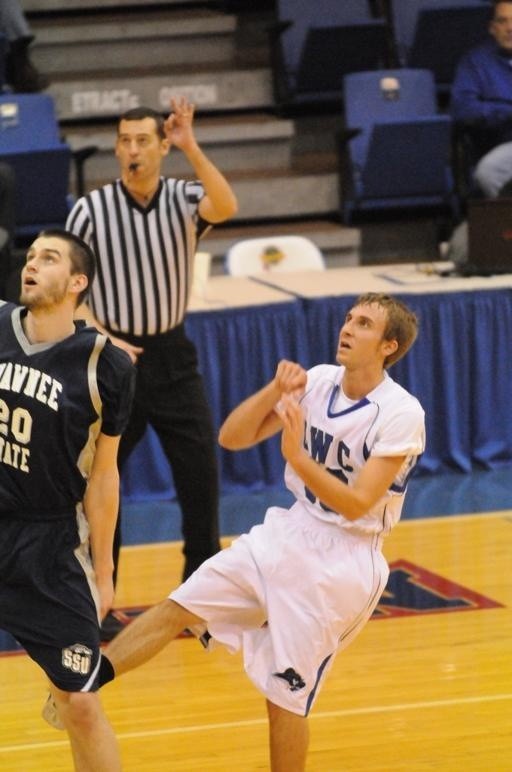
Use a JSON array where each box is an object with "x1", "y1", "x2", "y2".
[
  {"x1": 252, "y1": 269, "x2": 509, "y2": 478},
  {"x1": 147, "y1": 275, "x2": 305, "y2": 493}
]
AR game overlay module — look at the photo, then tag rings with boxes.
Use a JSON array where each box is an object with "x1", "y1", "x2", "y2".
[{"x1": 182, "y1": 113, "x2": 188, "y2": 117}]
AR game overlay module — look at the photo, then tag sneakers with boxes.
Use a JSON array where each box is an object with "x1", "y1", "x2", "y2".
[{"x1": 42, "y1": 694, "x2": 66, "y2": 731}]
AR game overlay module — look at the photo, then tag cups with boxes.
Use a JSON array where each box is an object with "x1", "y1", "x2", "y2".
[{"x1": 194, "y1": 253, "x2": 210, "y2": 282}]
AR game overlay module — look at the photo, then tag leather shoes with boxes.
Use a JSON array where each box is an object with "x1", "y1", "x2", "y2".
[
  {"x1": 4, "y1": 51, "x2": 50, "y2": 92},
  {"x1": 100, "y1": 617, "x2": 125, "y2": 642}
]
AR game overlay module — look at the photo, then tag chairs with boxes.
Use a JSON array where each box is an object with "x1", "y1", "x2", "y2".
[
  {"x1": 230, "y1": 3, "x2": 510, "y2": 268},
  {"x1": 2, "y1": 1, "x2": 92, "y2": 273}
]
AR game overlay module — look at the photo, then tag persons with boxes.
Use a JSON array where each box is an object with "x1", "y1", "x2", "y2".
[
  {"x1": 63, "y1": 95, "x2": 241, "y2": 654},
  {"x1": 0, "y1": 227, "x2": 148, "y2": 771},
  {"x1": 42, "y1": 290, "x2": 426, "y2": 772},
  {"x1": 0, "y1": 0, "x2": 51, "y2": 94},
  {"x1": 449, "y1": 1, "x2": 511, "y2": 209}
]
